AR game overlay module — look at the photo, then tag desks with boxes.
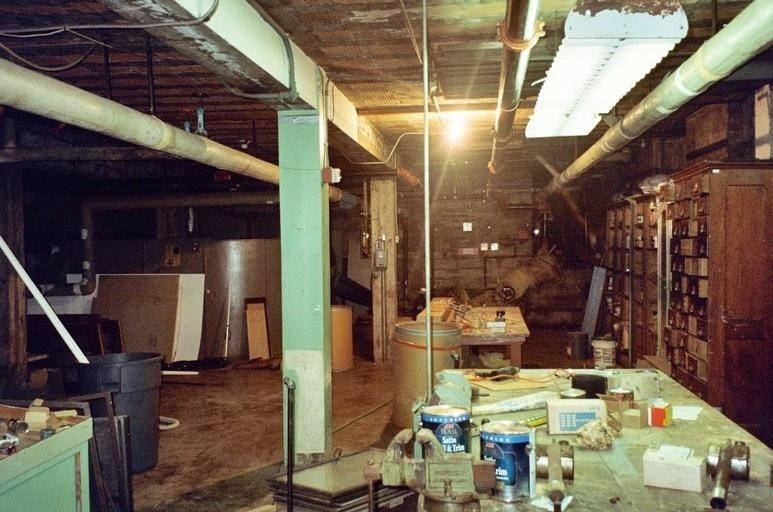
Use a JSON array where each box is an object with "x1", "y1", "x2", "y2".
[
  {"x1": 458, "y1": 306, "x2": 530, "y2": 368},
  {"x1": 441, "y1": 368, "x2": 772, "y2": 511}
]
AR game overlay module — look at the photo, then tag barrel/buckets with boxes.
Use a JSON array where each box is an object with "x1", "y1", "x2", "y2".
[
  {"x1": 590, "y1": 335, "x2": 619, "y2": 369},
  {"x1": 72, "y1": 350, "x2": 165, "y2": 475},
  {"x1": 566, "y1": 331, "x2": 589, "y2": 359},
  {"x1": 331, "y1": 304, "x2": 355, "y2": 373},
  {"x1": 477, "y1": 418, "x2": 538, "y2": 502},
  {"x1": 417, "y1": 404, "x2": 470, "y2": 452},
  {"x1": 391, "y1": 320, "x2": 463, "y2": 428}
]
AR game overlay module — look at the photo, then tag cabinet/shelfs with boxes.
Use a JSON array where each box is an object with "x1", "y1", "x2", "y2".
[
  {"x1": 666, "y1": 161, "x2": 773, "y2": 444},
  {"x1": 630, "y1": 180, "x2": 666, "y2": 369},
  {"x1": 599, "y1": 198, "x2": 632, "y2": 369},
  {"x1": 1, "y1": 403, "x2": 94, "y2": 511}
]
